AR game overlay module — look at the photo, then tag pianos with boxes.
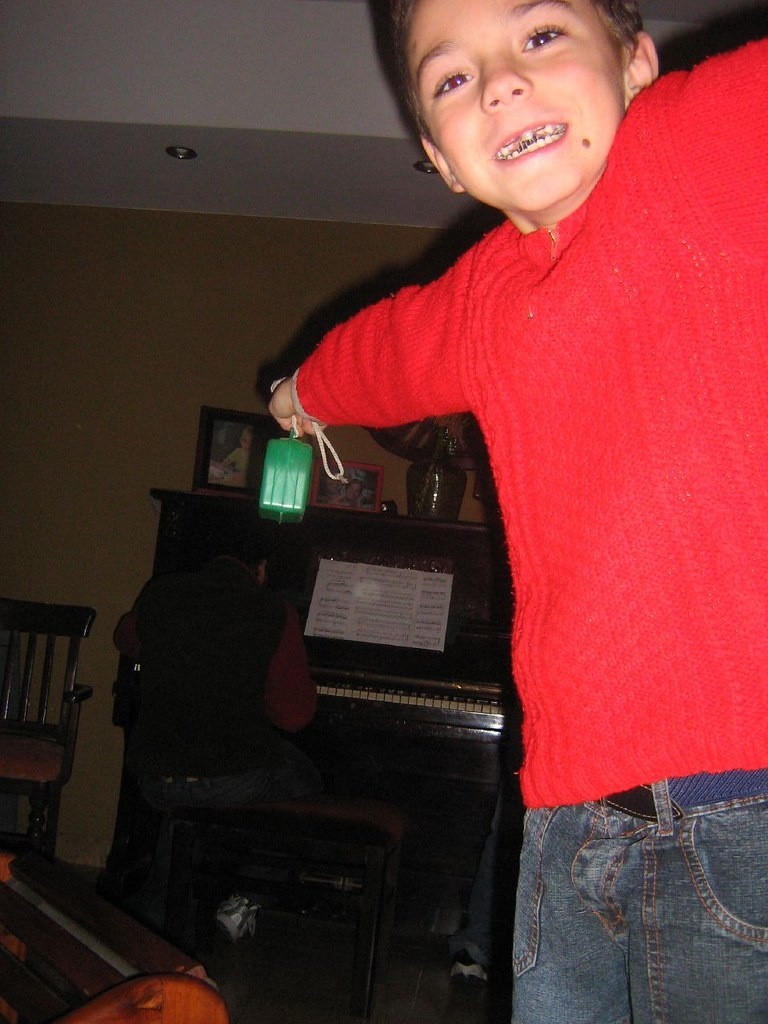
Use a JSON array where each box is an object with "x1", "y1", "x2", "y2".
[{"x1": 101, "y1": 481, "x2": 529, "y2": 1001}]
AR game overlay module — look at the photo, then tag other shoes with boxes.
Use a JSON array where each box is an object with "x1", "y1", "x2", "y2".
[
  {"x1": 450, "y1": 959, "x2": 495, "y2": 994},
  {"x1": 215, "y1": 894, "x2": 259, "y2": 945}
]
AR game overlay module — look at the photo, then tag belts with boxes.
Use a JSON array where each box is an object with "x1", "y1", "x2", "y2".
[{"x1": 600, "y1": 783, "x2": 685, "y2": 824}]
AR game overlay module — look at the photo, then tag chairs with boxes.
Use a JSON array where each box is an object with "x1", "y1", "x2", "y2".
[{"x1": 0, "y1": 596, "x2": 96, "y2": 856}]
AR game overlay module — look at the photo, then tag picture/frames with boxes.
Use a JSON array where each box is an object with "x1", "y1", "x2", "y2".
[
  {"x1": 310, "y1": 457, "x2": 384, "y2": 513},
  {"x1": 191, "y1": 405, "x2": 290, "y2": 497}
]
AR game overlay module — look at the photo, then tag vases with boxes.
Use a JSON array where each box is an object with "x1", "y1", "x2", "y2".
[{"x1": 406, "y1": 426, "x2": 467, "y2": 521}]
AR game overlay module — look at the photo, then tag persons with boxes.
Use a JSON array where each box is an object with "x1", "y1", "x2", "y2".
[
  {"x1": 112, "y1": 513, "x2": 325, "y2": 812},
  {"x1": 210, "y1": 422, "x2": 259, "y2": 482},
  {"x1": 269, "y1": 0, "x2": 768, "y2": 1024},
  {"x1": 320, "y1": 466, "x2": 368, "y2": 509}
]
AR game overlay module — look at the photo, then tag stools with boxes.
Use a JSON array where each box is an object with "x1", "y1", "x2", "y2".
[{"x1": 163, "y1": 793, "x2": 414, "y2": 1024}]
